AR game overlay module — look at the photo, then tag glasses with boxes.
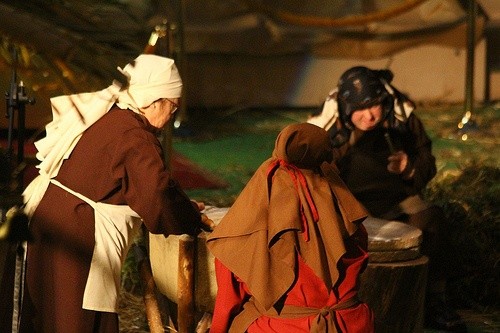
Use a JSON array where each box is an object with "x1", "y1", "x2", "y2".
[{"x1": 164, "y1": 98, "x2": 180, "y2": 114}]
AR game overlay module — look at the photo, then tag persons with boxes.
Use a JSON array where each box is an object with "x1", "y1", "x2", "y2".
[
  {"x1": 11, "y1": 51, "x2": 213, "y2": 332},
  {"x1": 203, "y1": 118, "x2": 377, "y2": 333},
  {"x1": 304, "y1": 65, "x2": 468, "y2": 332}
]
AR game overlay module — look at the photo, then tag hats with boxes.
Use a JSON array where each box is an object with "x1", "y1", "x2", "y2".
[
  {"x1": 34, "y1": 54, "x2": 183, "y2": 180},
  {"x1": 339, "y1": 66, "x2": 394, "y2": 120}
]
